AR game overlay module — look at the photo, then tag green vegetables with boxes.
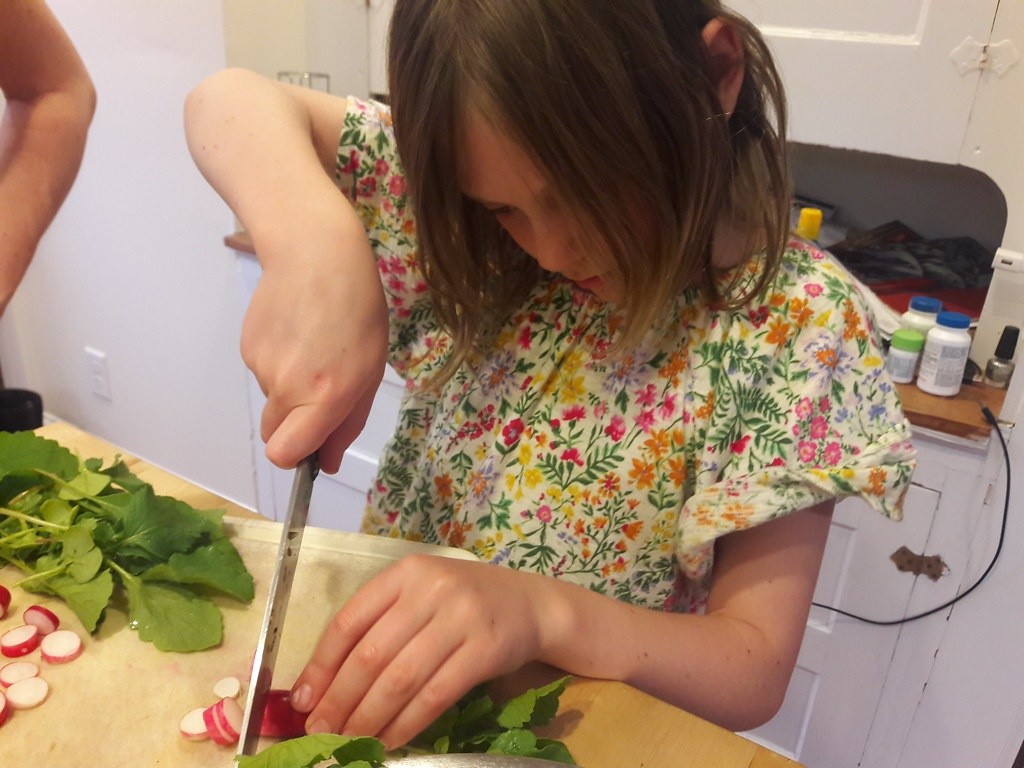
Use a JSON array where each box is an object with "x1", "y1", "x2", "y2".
[{"x1": 0, "y1": 430, "x2": 257, "y2": 652}]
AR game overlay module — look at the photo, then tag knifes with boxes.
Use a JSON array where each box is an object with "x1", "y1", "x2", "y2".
[{"x1": 235, "y1": 449, "x2": 321, "y2": 768}]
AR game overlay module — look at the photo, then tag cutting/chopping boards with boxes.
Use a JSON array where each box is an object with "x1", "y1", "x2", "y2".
[{"x1": 1, "y1": 515, "x2": 483, "y2": 768}]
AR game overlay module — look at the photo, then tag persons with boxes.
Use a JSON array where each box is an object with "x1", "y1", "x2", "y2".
[
  {"x1": 183, "y1": 0, "x2": 916, "y2": 755},
  {"x1": 0, "y1": 0, "x2": 97, "y2": 318}
]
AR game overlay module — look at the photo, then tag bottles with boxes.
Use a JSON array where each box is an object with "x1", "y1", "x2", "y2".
[
  {"x1": 798, "y1": 208, "x2": 823, "y2": 253},
  {"x1": 885, "y1": 329, "x2": 924, "y2": 384},
  {"x1": 899, "y1": 295, "x2": 943, "y2": 376},
  {"x1": 916, "y1": 311, "x2": 972, "y2": 396}
]
